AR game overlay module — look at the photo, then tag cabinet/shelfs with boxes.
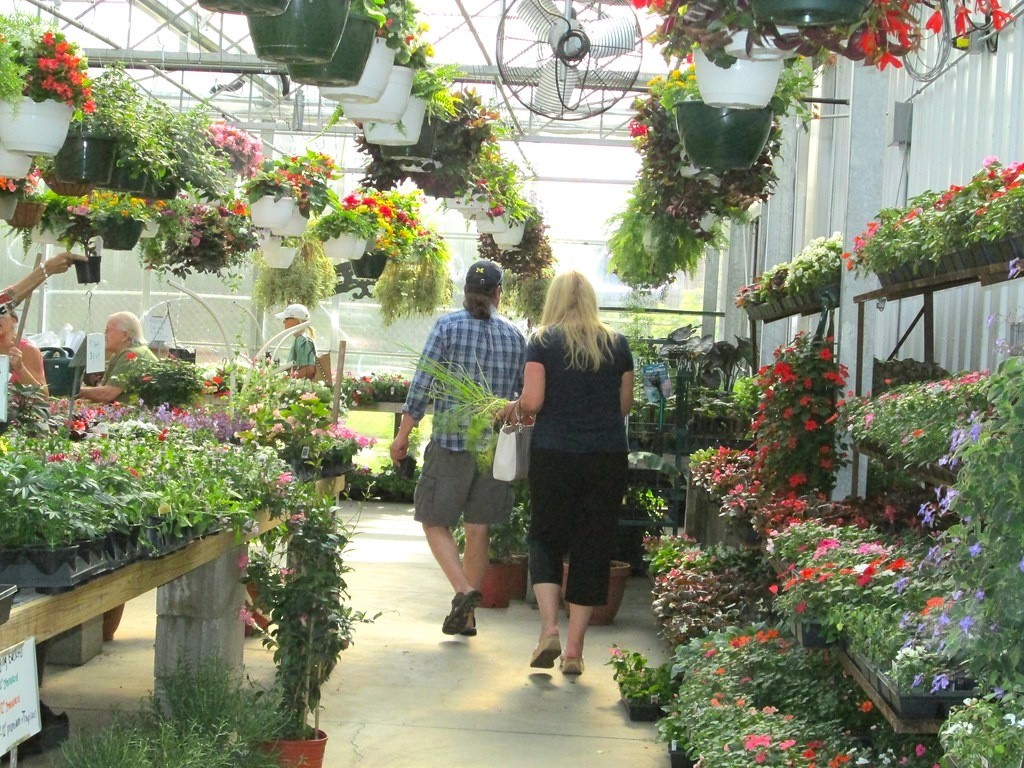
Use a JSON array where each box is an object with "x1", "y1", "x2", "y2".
[{"x1": 832, "y1": 257, "x2": 1024, "y2": 733}]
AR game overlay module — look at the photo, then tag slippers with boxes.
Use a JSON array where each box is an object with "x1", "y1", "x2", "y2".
[
  {"x1": 530, "y1": 637, "x2": 561, "y2": 668},
  {"x1": 560, "y1": 657, "x2": 585, "y2": 675},
  {"x1": 460, "y1": 590, "x2": 483, "y2": 636},
  {"x1": 442, "y1": 592, "x2": 472, "y2": 636}
]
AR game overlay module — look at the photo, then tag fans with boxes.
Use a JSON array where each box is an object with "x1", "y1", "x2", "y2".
[{"x1": 495, "y1": 0, "x2": 643, "y2": 122}]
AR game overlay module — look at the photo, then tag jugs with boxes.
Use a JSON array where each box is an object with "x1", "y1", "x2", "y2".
[{"x1": 38, "y1": 346, "x2": 85, "y2": 396}]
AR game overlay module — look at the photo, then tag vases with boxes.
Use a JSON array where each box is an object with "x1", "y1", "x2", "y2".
[
  {"x1": 7, "y1": 203, "x2": 48, "y2": 227},
  {"x1": 0, "y1": 195, "x2": 18, "y2": 220},
  {"x1": 323, "y1": 232, "x2": 368, "y2": 261},
  {"x1": 0, "y1": 96, "x2": 74, "y2": 155},
  {"x1": 320, "y1": 37, "x2": 401, "y2": 104},
  {"x1": 285, "y1": 14, "x2": 381, "y2": 87},
  {"x1": 266, "y1": 246, "x2": 297, "y2": 270},
  {"x1": 270, "y1": 206, "x2": 309, "y2": 236},
  {"x1": 102, "y1": 217, "x2": 144, "y2": 250},
  {"x1": 250, "y1": 728, "x2": 328, "y2": 768},
  {"x1": 197, "y1": 0, "x2": 290, "y2": 17},
  {"x1": 74, "y1": 255, "x2": 101, "y2": 284},
  {"x1": 1, "y1": 144, "x2": 32, "y2": 179},
  {"x1": 340, "y1": 66, "x2": 414, "y2": 122},
  {"x1": 362, "y1": 99, "x2": 429, "y2": 146},
  {"x1": 251, "y1": 196, "x2": 296, "y2": 230},
  {"x1": 352, "y1": 252, "x2": 388, "y2": 279},
  {"x1": 673, "y1": 100, "x2": 773, "y2": 171},
  {"x1": 54, "y1": 132, "x2": 117, "y2": 183},
  {"x1": 694, "y1": 46, "x2": 783, "y2": 109},
  {"x1": 247, "y1": 0, "x2": 351, "y2": 64}
]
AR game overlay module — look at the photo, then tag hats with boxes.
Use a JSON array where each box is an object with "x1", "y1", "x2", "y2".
[
  {"x1": 275, "y1": 304, "x2": 310, "y2": 322},
  {"x1": 466, "y1": 260, "x2": 503, "y2": 292}
]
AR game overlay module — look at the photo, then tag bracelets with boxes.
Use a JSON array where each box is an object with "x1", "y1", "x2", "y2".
[
  {"x1": 514, "y1": 406, "x2": 523, "y2": 420},
  {"x1": 40, "y1": 262, "x2": 49, "y2": 292}
]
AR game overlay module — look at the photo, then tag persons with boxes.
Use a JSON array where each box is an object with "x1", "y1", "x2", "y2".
[
  {"x1": 389, "y1": 260, "x2": 526, "y2": 635},
  {"x1": 75, "y1": 313, "x2": 160, "y2": 410},
  {"x1": 275, "y1": 304, "x2": 318, "y2": 381},
  {"x1": 505, "y1": 272, "x2": 634, "y2": 673},
  {"x1": 0, "y1": 251, "x2": 88, "y2": 400}
]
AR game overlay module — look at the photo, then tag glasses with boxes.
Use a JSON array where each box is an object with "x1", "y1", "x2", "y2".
[
  {"x1": 285, "y1": 318, "x2": 293, "y2": 320},
  {"x1": 105, "y1": 328, "x2": 115, "y2": 333}
]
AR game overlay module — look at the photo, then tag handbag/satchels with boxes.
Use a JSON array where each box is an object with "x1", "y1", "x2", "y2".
[
  {"x1": 285, "y1": 334, "x2": 332, "y2": 388},
  {"x1": 493, "y1": 400, "x2": 534, "y2": 483}
]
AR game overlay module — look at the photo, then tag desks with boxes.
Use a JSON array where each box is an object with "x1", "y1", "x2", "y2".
[{"x1": 0, "y1": 475, "x2": 345, "y2": 719}]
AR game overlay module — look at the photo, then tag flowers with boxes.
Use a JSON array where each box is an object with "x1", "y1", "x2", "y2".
[{"x1": 0, "y1": 0, "x2": 1023, "y2": 768}]
